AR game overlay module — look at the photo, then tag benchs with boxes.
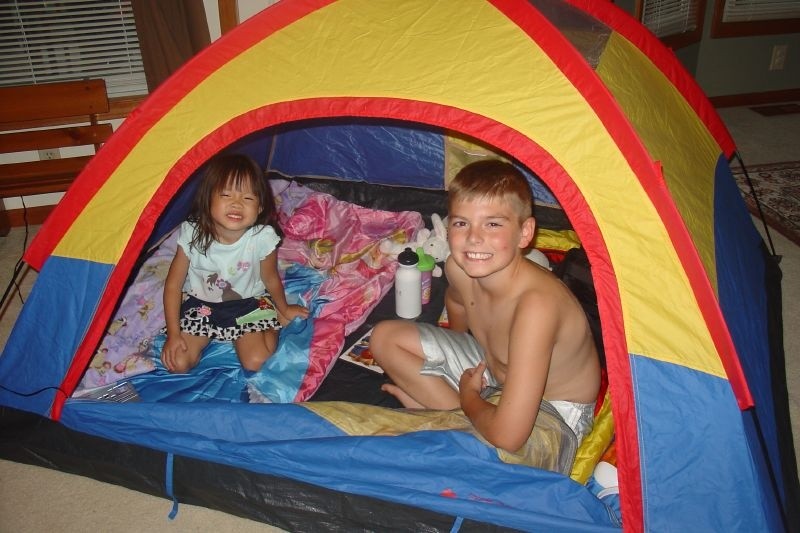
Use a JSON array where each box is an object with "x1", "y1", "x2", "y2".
[{"x1": 0, "y1": 79, "x2": 113, "y2": 225}]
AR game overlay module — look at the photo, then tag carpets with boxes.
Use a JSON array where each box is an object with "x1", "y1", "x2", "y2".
[{"x1": 730, "y1": 160, "x2": 800, "y2": 245}]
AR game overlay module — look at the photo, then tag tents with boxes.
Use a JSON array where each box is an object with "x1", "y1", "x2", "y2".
[{"x1": 0, "y1": 0, "x2": 783, "y2": 533}]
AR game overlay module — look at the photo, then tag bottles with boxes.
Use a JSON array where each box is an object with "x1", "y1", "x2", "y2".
[
  {"x1": 395, "y1": 247, "x2": 422, "y2": 319},
  {"x1": 415, "y1": 247, "x2": 435, "y2": 304}
]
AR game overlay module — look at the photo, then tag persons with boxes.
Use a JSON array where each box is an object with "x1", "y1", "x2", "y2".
[
  {"x1": 370, "y1": 159, "x2": 602, "y2": 453},
  {"x1": 160, "y1": 154, "x2": 312, "y2": 375}
]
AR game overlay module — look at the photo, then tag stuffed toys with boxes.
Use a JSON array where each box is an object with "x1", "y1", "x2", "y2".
[{"x1": 379, "y1": 214, "x2": 451, "y2": 277}]
